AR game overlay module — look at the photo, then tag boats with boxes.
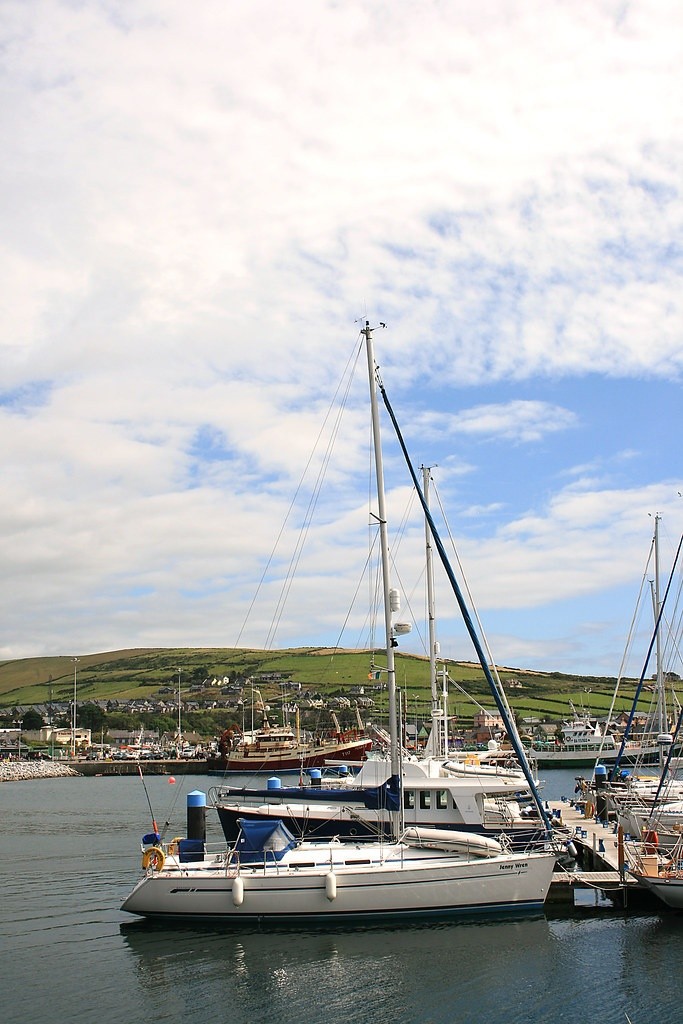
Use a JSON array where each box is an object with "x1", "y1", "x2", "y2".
[
  {"x1": 219, "y1": 717, "x2": 374, "y2": 774},
  {"x1": 211, "y1": 757, "x2": 553, "y2": 861},
  {"x1": 64, "y1": 756, "x2": 209, "y2": 776},
  {"x1": 511, "y1": 713, "x2": 683, "y2": 770}
]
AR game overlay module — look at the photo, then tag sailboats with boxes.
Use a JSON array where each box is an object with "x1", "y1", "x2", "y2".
[
  {"x1": 576, "y1": 504, "x2": 683, "y2": 904},
  {"x1": 113, "y1": 318, "x2": 563, "y2": 928},
  {"x1": 355, "y1": 464, "x2": 546, "y2": 794}
]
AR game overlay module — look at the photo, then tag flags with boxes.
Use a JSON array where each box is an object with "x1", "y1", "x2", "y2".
[{"x1": 368, "y1": 672, "x2": 380, "y2": 680}]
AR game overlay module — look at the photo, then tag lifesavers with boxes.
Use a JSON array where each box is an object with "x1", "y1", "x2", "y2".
[
  {"x1": 142, "y1": 847, "x2": 164, "y2": 871},
  {"x1": 168, "y1": 837, "x2": 185, "y2": 854}
]
